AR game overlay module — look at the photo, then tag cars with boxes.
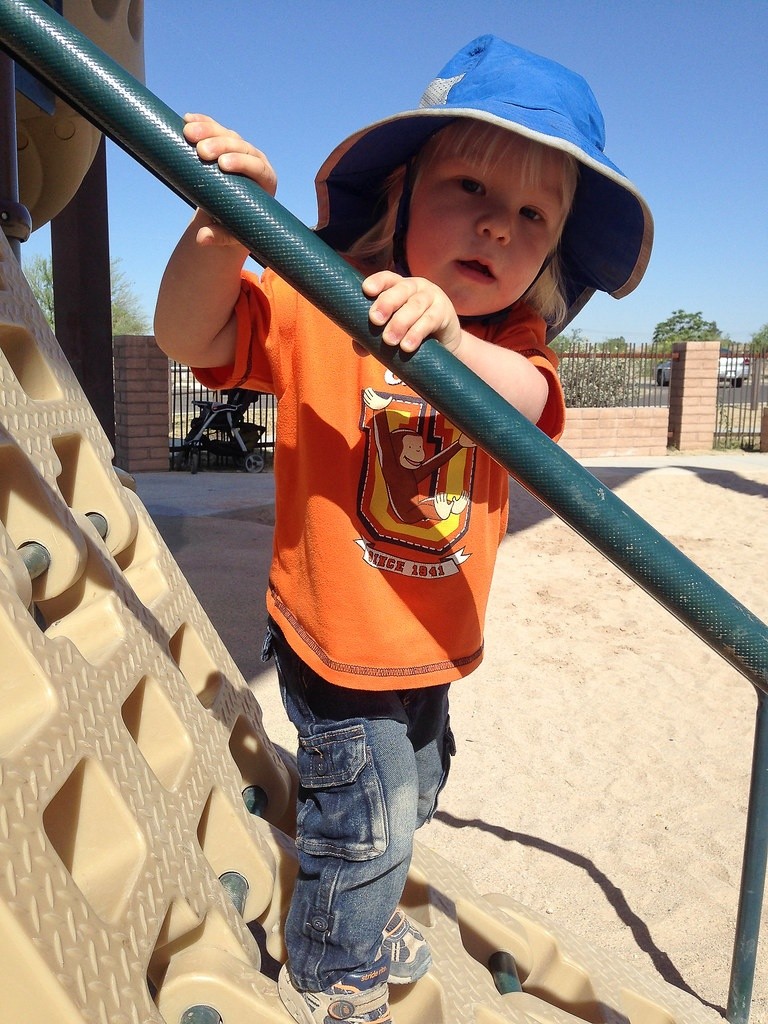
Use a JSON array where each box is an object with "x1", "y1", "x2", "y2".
[{"x1": 655, "y1": 348, "x2": 750, "y2": 387}]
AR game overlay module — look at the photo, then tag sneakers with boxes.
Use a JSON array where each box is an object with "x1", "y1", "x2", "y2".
[{"x1": 278, "y1": 909, "x2": 433, "y2": 1024}]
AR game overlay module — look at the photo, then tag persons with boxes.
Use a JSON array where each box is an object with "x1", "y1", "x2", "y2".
[{"x1": 153, "y1": 35, "x2": 654, "y2": 1024}]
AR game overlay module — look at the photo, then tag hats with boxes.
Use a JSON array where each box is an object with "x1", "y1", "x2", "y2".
[{"x1": 304, "y1": 36, "x2": 655, "y2": 343}]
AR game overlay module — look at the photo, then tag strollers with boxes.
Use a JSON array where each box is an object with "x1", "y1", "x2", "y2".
[{"x1": 176, "y1": 388, "x2": 266, "y2": 474}]
